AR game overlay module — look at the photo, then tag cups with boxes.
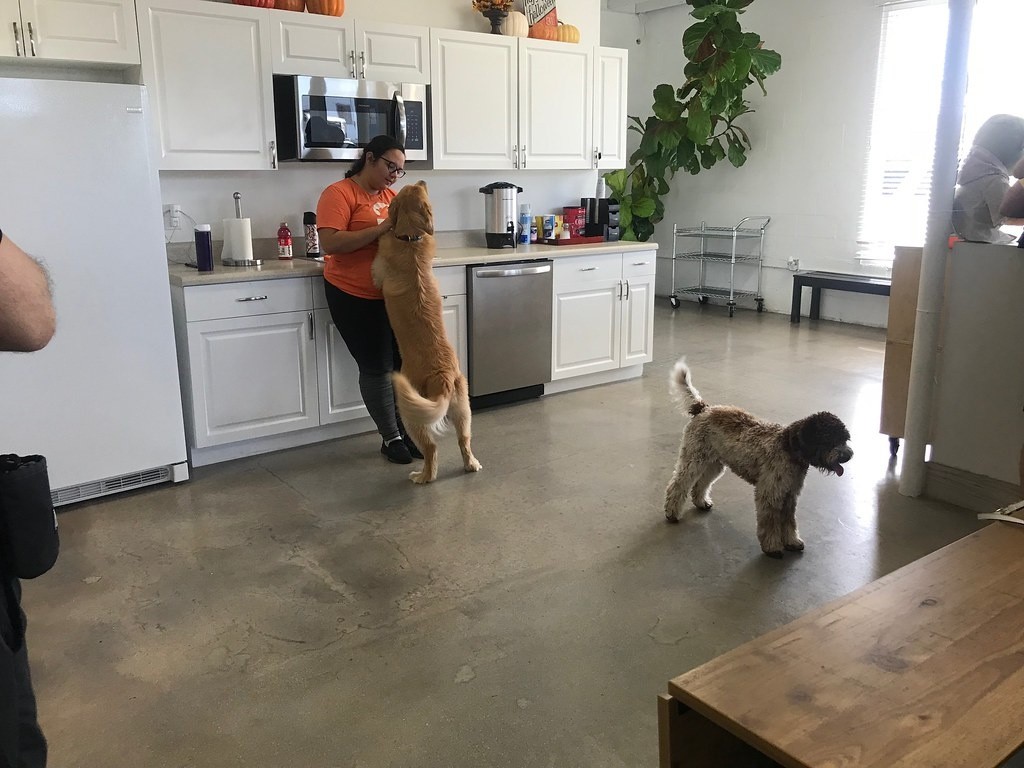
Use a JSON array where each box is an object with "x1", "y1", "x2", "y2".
[{"x1": 597, "y1": 178, "x2": 605, "y2": 199}]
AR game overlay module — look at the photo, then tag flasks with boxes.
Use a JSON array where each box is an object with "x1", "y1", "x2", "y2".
[{"x1": 194, "y1": 224, "x2": 213, "y2": 272}]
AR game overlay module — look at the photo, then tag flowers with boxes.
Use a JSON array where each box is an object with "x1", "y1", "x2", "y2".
[{"x1": 472, "y1": 0, "x2": 514, "y2": 12}]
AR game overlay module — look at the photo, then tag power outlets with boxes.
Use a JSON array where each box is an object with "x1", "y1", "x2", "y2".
[{"x1": 163, "y1": 203, "x2": 181, "y2": 231}]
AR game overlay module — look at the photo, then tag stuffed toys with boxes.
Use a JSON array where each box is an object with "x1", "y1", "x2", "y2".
[{"x1": 951, "y1": 114, "x2": 1024, "y2": 247}]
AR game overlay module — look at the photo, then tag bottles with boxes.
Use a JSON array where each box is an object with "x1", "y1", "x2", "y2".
[
  {"x1": 277, "y1": 222, "x2": 293, "y2": 260},
  {"x1": 519, "y1": 204, "x2": 531, "y2": 247},
  {"x1": 303, "y1": 211, "x2": 320, "y2": 258},
  {"x1": 560, "y1": 224, "x2": 570, "y2": 239}
]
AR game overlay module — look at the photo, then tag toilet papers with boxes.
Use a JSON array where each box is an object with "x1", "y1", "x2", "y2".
[{"x1": 219, "y1": 217, "x2": 254, "y2": 261}]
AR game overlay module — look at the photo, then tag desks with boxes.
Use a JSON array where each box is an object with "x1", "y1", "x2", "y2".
[{"x1": 659, "y1": 510, "x2": 1024, "y2": 767}]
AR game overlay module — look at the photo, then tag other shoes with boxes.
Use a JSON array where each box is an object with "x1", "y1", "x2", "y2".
[
  {"x1": 381, "y1": 438, "x2": 413, "y2": 464},
  {"x1": 403, "y1": 435, "x2": 425, "y2": 459}
]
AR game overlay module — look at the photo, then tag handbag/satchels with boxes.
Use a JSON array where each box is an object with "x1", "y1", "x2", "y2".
[{"x1": 0, "y1": 453, "x2": 60, "y2": 580}]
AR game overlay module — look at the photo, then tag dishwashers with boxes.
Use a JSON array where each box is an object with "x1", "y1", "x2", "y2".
[{"x1": 468, "y1": 260, "x2": 553, "y2": 409}]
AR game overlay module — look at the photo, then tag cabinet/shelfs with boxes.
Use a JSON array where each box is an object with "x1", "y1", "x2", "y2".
[
  {"x1": 0, "y1": 1, "x2": 140, "y2": 74},
  {"x1": 544, "y1": 250, "x2": 662, "y2": 395},
  {"x1": 670, "y1": 216, "x2": 773, "y2": 319},
  {"x1": 596, "y1": 47, "x2": 630, "y2": 171},
  {"x1": 279, "y1": 10, "x2": 431, "y2": 83},
  {"x1": 436, "y1": 29, "x2": 596, "y2": 173},
  {"x1": 137, "y1": 2, "x2": 279, "y2": 172},
  {"x1": 183, "y1": 265, "x2": 469, "y2": 449}
]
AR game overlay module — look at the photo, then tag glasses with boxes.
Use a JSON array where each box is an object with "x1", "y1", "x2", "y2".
[{"x1": 374, "y1": 153, "x2": 406, "y2": 178}]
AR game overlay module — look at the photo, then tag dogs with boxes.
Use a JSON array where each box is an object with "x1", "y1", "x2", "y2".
[
  {"x1": 662, "y1": 352, "x2": 856, "y2": 561},
  {"x1": 367, "y1": 177, "x2": 484, "y2": 488}
]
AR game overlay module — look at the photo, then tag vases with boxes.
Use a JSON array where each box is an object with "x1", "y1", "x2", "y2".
[{"x1": 483, "y1": 10, "x2": 509, "y2": 34}]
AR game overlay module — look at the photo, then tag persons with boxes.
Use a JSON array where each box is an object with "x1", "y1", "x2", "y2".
[
  {"x1": 318, "y1": 135, "x2": 429, "y2": 463},
  {"x1": 0, "y1": 228, "x2": 62, "y2": 768}
]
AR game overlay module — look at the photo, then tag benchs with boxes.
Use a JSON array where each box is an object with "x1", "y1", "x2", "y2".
[{"x1": 786, "y1": 268, "x2": 893, "y2": 325}]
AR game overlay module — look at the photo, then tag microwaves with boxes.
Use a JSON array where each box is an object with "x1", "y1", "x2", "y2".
[{"x1": 275, "y1": 76, "x2": 427, "y2": 162}]
